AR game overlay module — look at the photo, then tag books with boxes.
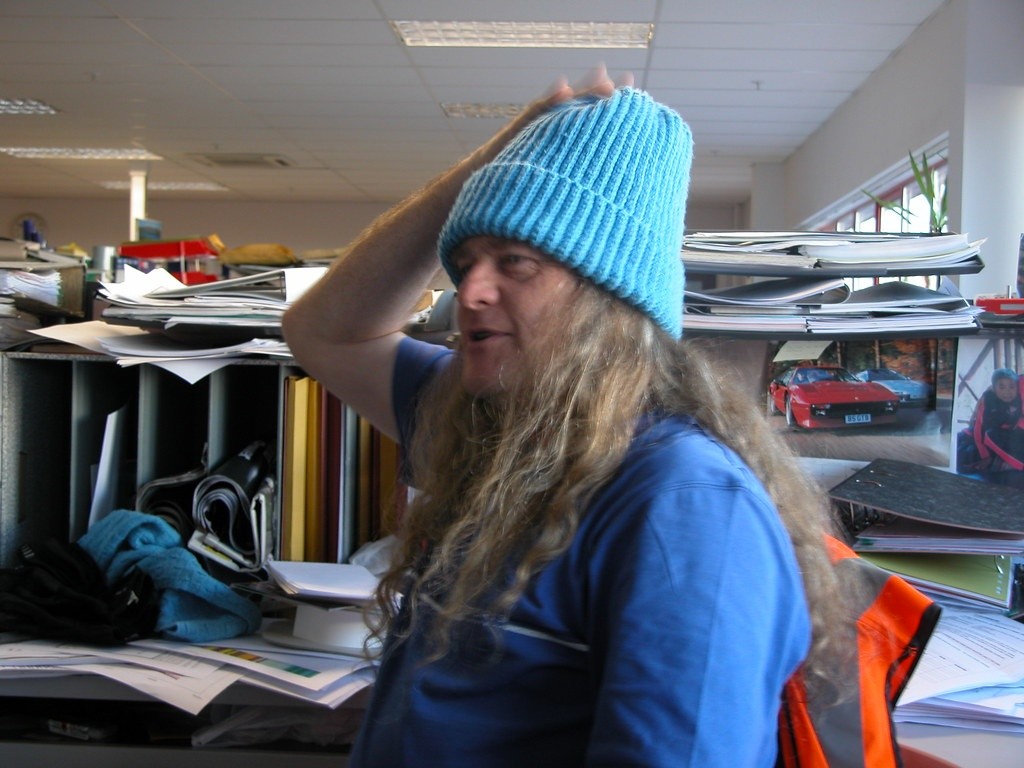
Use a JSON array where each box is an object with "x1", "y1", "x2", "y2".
[
  {"x1": 0, "y1": 559, "x2": 403, "y2": 715},
  {"x1": 279, "y1": 64, "x2": 861, "y2": 768},
  {"x1": 668, "y1": 230, "x2": 986, "y2": 334},
  {"x1": 0, "y1": 236, "x2": 330, "y2": 384}
]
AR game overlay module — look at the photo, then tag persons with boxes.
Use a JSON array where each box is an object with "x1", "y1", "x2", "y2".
[{"x1": 957, "y1": 367, "x2": 1024, "y2": 491}]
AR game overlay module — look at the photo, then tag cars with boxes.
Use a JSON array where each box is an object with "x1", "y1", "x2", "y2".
[
  {"x1": 855, "y1": 368, "x2": 928, "y2": 408},
  {"x1": 766, "y1": 361, "x2": 900, "y2": 431}
]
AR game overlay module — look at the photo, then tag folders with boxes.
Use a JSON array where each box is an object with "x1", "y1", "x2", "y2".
[{"x1": 278, "y1": 375, "x2": 408, "y2": 564}]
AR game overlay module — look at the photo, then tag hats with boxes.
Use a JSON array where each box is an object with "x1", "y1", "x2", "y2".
[{"x1": 436, "y1": 86, "x2": 693, "y2": 344}]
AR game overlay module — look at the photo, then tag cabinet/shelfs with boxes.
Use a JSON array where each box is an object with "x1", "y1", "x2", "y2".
[{"x1": 680, "y1": 228, "x2": 983, "y2": 342}]
